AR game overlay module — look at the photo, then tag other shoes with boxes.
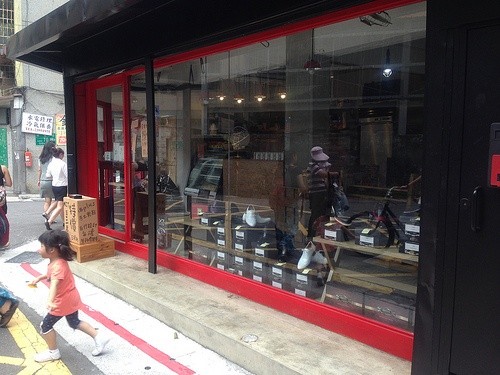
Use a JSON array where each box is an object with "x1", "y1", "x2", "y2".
[
  {"x1": 42, "y1": 211, "x2": 49, "y2": 218},
  {"x1": 0, "y1": 298, "x2": 20, "y2": 327},
  {"x1": 52, "y1": 220, "x2": 57, "y2": 223}
]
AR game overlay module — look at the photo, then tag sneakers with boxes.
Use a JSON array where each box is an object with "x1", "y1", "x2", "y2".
[
  {"x1": 242, "y1": 211, "x2": 272, "y2": 224},
  {"x1": 90, "y1": 335, "x2": 110, "y2": 356},
  {"x1": 310, "y1": 250, "x2": 328, "y2": 264},
  {"x1": 297, "y1": 241, "x2": 316, "y2": 269},
  {"x1": 245, "y1": 204, "x2": 256, "y2": 226},
  {"x1": 33, "y1": 347, "x2": 62, "y2": 362}
]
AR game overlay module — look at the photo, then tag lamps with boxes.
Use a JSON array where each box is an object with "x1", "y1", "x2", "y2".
[
  {"x1": 383, "y1": 48, "x2": 392, "y2": 77},
  {"x1": 12, "y1": 87, "x2": 25, "y2": 110}
]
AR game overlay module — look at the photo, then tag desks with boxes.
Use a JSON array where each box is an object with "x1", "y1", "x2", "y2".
[
  {"x1": 167, "y1": 216, "x2": 280, "y2": 275},
  {"x1": 313, "y1": 234, "x2": 420, "y2": 295}
]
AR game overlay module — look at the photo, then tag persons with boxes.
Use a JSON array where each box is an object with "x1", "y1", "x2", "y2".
[
  {"x1": 29, "y1": 230, "x2": 110, "y2": 363},
  {"x1": 44, "y1": 147, "x2": 67, "y2": 229},
  {"x1": 37, "y1": 140, "x2": 57, "y2": 224},
  {"x1": 258, "y1": 145, "x2": 421, "y2": 263},
  {"x1": 0, "y1": 287, "x2": 20, "y2": 327},
  {"x1": 0, "y1": 165, "x2": 12, "y2": 248}
]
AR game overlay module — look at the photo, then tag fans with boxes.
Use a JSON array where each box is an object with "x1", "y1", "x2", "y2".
[{"x1": 361, "y1": 11, "x2": 391, "y2": 28}]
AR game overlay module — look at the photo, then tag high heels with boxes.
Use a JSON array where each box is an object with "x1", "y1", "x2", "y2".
[{"x1": 44, "y1": 220, "x2": 52, "y2": 230}]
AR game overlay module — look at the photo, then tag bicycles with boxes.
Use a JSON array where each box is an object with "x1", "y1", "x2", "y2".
[{"x1": 344, "y1": 185, "x2": 419, "y2": 255}]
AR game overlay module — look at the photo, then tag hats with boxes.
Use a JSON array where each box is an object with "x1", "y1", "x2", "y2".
[{"x1": 310, "y1": 146, "x2": 329, "y2": 161}]
